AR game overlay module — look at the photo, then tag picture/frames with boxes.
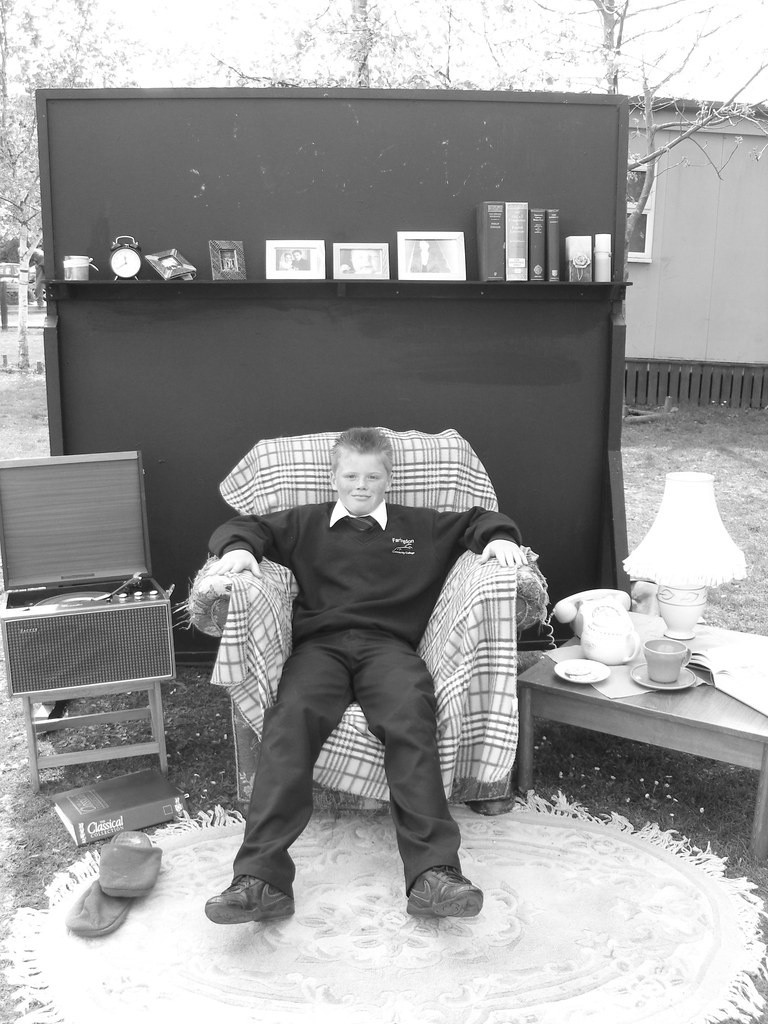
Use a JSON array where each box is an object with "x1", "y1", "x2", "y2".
[
  {"x1": 266, "y1": 240, "x2": 326, "y2": 279},
  {"x1": 209, "y1": 240, "x2": 247, "y2": 280},
  {"x1": 397, "y1": 231, "x2": 467, "y2": 281},
  {"x1": 332, "y1": 243, "x2": 390, "y2": 280},
  {"x1": 144, "y1": 248, "x2": 197, "y2": 280}
]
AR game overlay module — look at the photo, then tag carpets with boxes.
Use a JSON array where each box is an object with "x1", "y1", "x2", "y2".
[{"x1": 3, "y1": 791, "x2": 765, "y2": 1024}]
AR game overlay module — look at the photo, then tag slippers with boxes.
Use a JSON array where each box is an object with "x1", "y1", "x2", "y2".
[
  {"x1": 66, "y1": 879, "x2": 132, "y2": 937},
  {"x1": 98, "y1": 831, "x2": 163, "y2": 898}
]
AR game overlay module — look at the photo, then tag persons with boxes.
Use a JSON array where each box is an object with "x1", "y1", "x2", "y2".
[
  {"x1": 204, "y1": 426, "x2": 529, "y2": 925},
  {"x1": 279, "y1": 250, "x2": 310, "y2": 270},
  {"x1": 410, "y1": 241, "x2": 442, "y2": 274}
]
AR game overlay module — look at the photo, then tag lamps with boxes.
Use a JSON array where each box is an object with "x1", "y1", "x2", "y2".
[{"x1": 622, "y1": 473, "x2": 747, "y2": 640}]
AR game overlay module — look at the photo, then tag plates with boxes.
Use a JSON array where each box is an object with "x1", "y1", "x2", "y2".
[
  {"x1": 554, "y1": 658, "x2": 612, "y2": 683},
  {"x1": 631, "y1": 663, "x2": 697, "y2": 690}
]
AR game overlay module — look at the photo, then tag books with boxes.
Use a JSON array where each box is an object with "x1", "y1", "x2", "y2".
[
  {"x1": 689, "y1": 639, "x2": 767, "y2": 717},
  {"x1": 476, "y1": 200, "x2": 559, "y2": 281},
  {"x1": 47, "y1": 766, "x2": 189, "y2": 846}
]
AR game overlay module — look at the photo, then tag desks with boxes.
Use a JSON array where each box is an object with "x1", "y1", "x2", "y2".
[{"x1": 517, "y1": 615, "x2": 768, "y2": 858}]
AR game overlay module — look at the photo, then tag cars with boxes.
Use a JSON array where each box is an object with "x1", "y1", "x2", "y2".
[{"x1": 0, "y1": 263, "x2": 47, "y2": 304}]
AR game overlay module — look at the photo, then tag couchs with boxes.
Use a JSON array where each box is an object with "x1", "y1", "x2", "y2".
[{"x1": 187, "y1": 427, "x2": 549, "y2": 816}]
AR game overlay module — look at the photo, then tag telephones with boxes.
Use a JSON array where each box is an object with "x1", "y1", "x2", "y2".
[{"x1": 552, "y1": 589, "x2": 639, "y2": 666}]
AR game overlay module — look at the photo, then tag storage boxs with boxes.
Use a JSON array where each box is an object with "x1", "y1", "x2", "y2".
[{"x1": 0, "y1": 449, "x2": 180, "y2": 702}]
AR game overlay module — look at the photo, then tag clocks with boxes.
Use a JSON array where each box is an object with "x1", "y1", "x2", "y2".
[{"x1": 109, "y1": 235, "x2": 142, "y2": 280}]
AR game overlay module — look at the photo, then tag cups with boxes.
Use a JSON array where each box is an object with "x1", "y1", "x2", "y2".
[{"x1": 643, "y1": 638, "x2": 691, "y2": 684}]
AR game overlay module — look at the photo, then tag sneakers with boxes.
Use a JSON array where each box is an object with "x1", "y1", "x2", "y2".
[
  {"x1": 405, "y1": 865, "x2": 484, "y2": 918},
  {"x1": 206, "y1": 876, "x2": 294, "y2": 925}
]
